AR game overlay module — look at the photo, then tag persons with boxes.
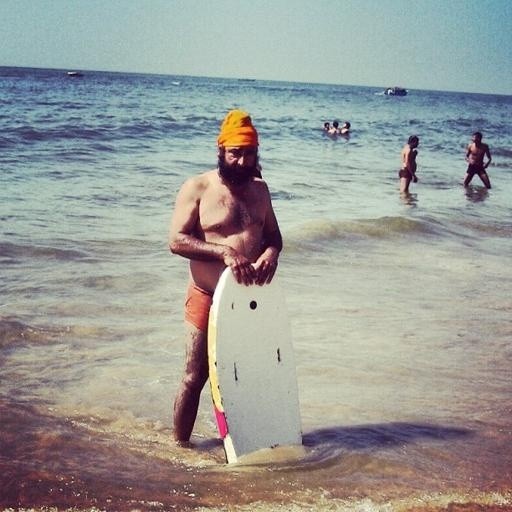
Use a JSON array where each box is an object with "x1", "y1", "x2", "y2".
[
  {"x1": 464, "y1": 133, "x2": 493, "y2": 189},
  {"x1": 169, "y1": 110, "x2": 285, "y2": 453},
  {"x1": 399, "y1": 193, "x2": 419, "y2": 209},
  {"x1": 324, "y1": 122, "x2": 331, "y2": 131},
  {"x1": 327, "y1": 122, "x2": 341, "y2": 135},
  {"x1": 340, "y1": 122, "x2": 350, "y2": 134},
  {"x1": 398, "y1": 135, "x2": 419, "y2": 195}
]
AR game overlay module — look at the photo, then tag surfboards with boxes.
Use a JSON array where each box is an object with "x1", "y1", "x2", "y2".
[{"x1": 208, "y1": 264, "x2": 304, "y2": 463}]
